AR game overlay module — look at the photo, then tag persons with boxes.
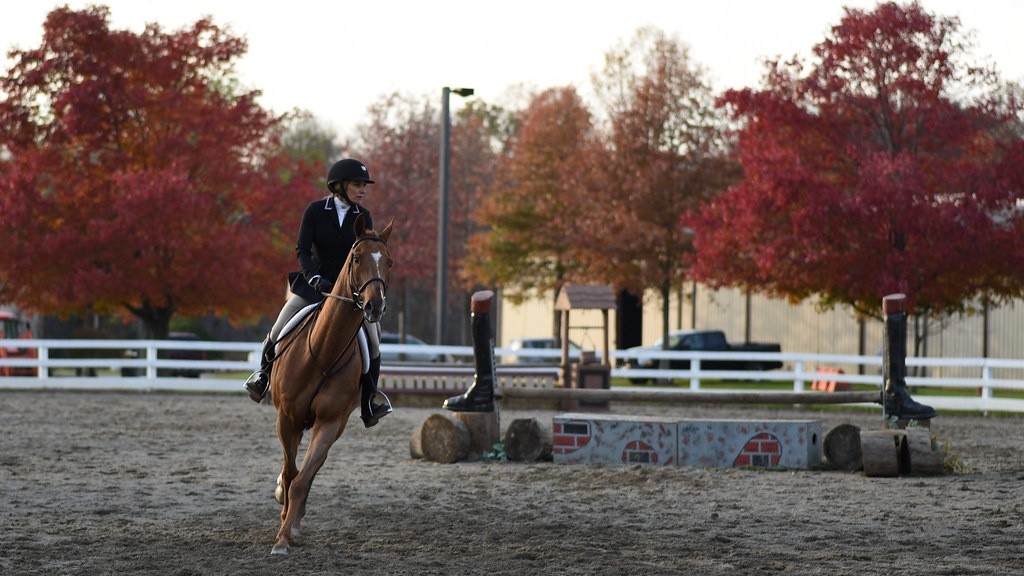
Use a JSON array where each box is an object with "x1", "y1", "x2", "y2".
[{"x1": 246, "y1": 159, "x2": 390, "y2": 428}]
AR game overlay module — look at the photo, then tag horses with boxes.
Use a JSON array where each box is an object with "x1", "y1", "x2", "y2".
[{"x1": 270, "y1": 212, "x2": 396, "y2": 556}]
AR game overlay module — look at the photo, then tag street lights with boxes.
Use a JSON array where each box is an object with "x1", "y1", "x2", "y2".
[{"x1": 436, "y1": 87, "x2": 473, "y2": 361}]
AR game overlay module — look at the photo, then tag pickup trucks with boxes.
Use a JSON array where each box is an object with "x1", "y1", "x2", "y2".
[{"x1": 623, "y1": 330, "x2": 783, "y2": 384}]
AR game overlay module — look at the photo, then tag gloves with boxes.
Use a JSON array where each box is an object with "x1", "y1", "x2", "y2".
[{"x1": 315, "y1": 277, "x2": 333, "y2": 293}]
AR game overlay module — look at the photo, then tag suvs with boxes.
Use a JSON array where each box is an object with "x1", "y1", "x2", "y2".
[{"x1": 121, "y1": 332, "x2": 206, "y2": 378}]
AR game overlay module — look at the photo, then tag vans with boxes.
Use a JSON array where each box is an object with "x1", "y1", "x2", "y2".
[{"x1": 511, "y1": 338, "x2": 584, "y2": 363}]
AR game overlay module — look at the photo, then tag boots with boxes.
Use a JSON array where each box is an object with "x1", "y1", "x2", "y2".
[
  {"x1": 360, "y1": 352, "x2": 390, "y2": 427},
  {"x1": 246, "y1": 333, "x2": 275, "y2": 403}
]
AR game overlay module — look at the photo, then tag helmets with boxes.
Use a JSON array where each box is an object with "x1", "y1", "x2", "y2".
[{"x1": 327, "y1": 159, "x2": 375, "y2": 186}]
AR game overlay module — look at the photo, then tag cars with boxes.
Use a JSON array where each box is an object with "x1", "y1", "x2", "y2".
[{"x1": 381, "y1": 333, "x2": 439, "y2": 362}]
